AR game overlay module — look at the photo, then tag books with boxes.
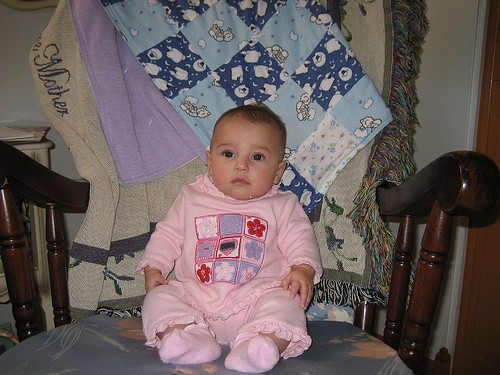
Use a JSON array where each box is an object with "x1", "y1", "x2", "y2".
[{"x1": 0, "y1": 124, "x2": 36, "y2": 140}]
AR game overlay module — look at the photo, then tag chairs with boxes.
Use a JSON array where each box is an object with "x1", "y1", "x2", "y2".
[{"x1": 0, "y1": 139, "x2": 500, "y2": 375}]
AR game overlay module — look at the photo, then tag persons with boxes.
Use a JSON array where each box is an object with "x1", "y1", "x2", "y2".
[{"x1": 136, "y1": 104, "x2": 324, "y2": 374}]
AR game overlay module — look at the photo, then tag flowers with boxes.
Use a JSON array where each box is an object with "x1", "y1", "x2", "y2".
[
  {"x1": 198, "y1": 218, "x2": 216, "y2": 237},
  {"x1": 221, "y1": 217, "x2": 239, "y2": 234},
  {"x1": 199, "y1": 242, "x2": 214, "y2": 258},
  {"x1": 244, "y1": 241, "x2": 263, "y2": 260},
  {"x1": 239, "y1": 266, "x2": 256, "y2": 284},
  {"x1": 197, "y1": 264, "x2": 210, "y2": 283},
  {"x1": 247, "y1": 219, "x2": 266, "y2": 237},
  {"x1": 214, "y1": 262, "x2": 235, "y2": 282}
]
